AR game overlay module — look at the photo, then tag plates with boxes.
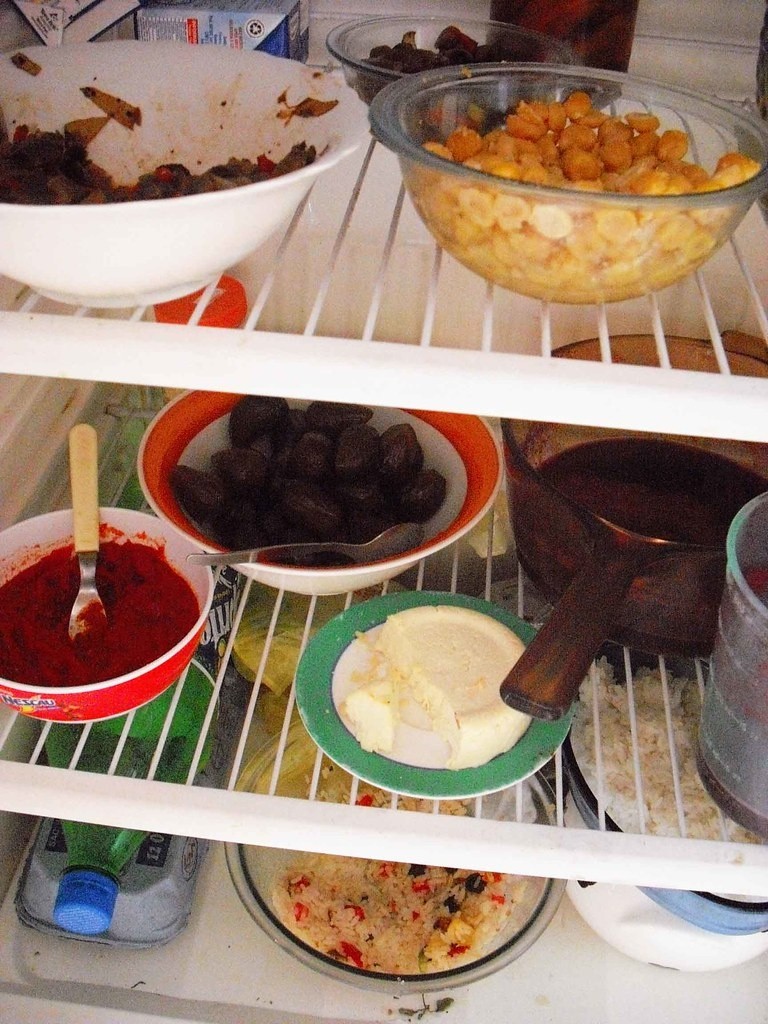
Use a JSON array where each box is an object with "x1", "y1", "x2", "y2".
[
  {"x1": 295, "y1": 591, "x2": 576, "y2": 799},
  {"x1": 225, "y1": 721, "x2": 570, "y2": 996}
]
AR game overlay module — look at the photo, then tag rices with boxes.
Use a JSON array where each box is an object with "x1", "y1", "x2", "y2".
[
  {"x1": 576, "y1": 655, "x2": 760, "y2": 844},
  {"x1": 273, "y1": 765, "x2": 524, "y2": 973}
]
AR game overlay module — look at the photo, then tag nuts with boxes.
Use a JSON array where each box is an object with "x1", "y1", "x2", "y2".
[{"x1": 415, "y1": 91, "x2": 761, "y2": 305}]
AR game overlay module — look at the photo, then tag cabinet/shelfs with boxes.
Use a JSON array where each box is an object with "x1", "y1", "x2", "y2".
[{"x1": 0, "y1": 137, "x2": 768, "y2": 909}]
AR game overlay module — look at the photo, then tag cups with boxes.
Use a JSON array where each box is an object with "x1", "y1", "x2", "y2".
[{"x1": 695, "y1": 492, "x2": 768, "y2": 841}]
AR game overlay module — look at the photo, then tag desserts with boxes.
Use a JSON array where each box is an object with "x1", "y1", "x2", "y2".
[{"x1": 341, "y1": 602, "x2": 534, "y2": 768}]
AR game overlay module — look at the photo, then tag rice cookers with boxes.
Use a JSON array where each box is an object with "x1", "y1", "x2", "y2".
[{"x1": 558, "y1": 732, "x2": 768, "y2": 973}]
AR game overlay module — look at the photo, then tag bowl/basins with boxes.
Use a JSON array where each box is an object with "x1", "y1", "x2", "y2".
[
  {"x1": 0, "y1": 39, "x2": 371, "y2": 308},
  {"x1": 325, "y1": 14, "x2": 583, "y2": 126},
  {"x1": 368, "y1": 63, "x2": 768, "y2": 304},
  {"x1": 136, "y1": 388, "x2": 505, "y2": 597},
  {"x1": 0, "y1": 506, "x2": 214, "y2": 724}
]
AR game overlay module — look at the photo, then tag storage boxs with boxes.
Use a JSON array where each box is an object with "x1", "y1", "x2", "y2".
[
  {"x1": 14, "y1": 0, "x2": 138, "y2": 45},
  {"x1": 131, "y1": 1, "x2": 310, "y2": 63}
]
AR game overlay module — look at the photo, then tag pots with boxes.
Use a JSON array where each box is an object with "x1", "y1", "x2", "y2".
[{"x1": 499, "y1": 327, "x2": 768, "y2": 719}]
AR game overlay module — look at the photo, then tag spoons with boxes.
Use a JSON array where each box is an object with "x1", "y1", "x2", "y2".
[
  {"x1": 68, "y1": 423, "x2": 107, "y2": 643},
  {"x1": 186, "y1": 523, "x2": 426, "y2": 567}
]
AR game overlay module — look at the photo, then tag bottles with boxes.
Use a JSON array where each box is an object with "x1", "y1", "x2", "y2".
[{"x1": 37, "y1": 551, "x2": 243, "y2": 934}]
